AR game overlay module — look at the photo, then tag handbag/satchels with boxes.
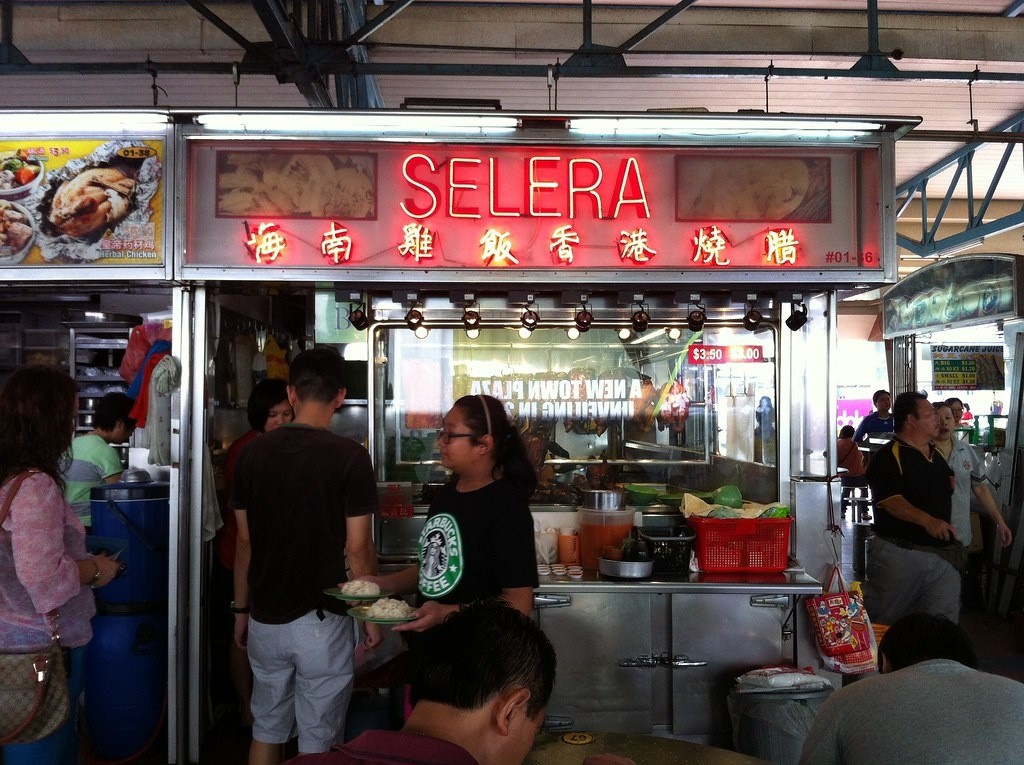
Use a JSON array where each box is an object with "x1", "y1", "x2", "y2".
[{"x1": 1, "y1": 642, "x2": 71, "y2": 744}]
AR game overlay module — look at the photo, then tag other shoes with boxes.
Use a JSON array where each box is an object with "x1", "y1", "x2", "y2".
[
  {"x1": 841, "y1": 510, "x2": 846, "y2": 518},
  {"x1": 863, "y1": 511, "x2": 872, "y2": 520}
]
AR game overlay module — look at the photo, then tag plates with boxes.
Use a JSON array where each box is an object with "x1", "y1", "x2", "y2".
[
  {"x1": 348, "y1": 605, "x2": 419, "y2": 624},
  {"x1": 324, "y1": 588, "x2": 395, "y2": 601}
]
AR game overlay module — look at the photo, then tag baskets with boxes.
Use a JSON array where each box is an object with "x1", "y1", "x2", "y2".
[
  {"x1": 687, "y1": 516, "x2": 796, "y2": 573},
  {"x1": 638, "y1": 524, "x2": 695, "y2": 571}
]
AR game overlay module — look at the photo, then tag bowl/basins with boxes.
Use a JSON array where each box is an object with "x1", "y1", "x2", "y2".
[
  {"x1": 537, "y1": 564, "x2": 583, "y2": 578},
  {"x1": 0, "y1": 150, "x2": 44, "y2": 266},
  {"x1": 79, "y1": 398, "x2": 99, "y2": 425}
]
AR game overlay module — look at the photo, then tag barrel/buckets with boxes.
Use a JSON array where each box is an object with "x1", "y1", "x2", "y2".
[
  {"x1": 576, "y1": 505, "x2": 636, "y2": 580},
  {"x1": 90, "y1": 481, "x2": 170, "y2": 602},
  {"x1": 91, "y1": 602, "x2": 167, "y2": 756},
  {"x1": 1, "y1": 647, "x2": 88, "y2": 765},
  {"x1": 700, "y1": 497, "x2": 714, "y2": 504},
  {"x1": 662, "y1": 499, "x2": 682, "y2": 506},
  {"x1": 624, "y1": 484, "x2": 658, "y2": 506}
]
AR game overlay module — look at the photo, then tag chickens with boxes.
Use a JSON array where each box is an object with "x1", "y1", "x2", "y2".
[{"x1": 450, "y1": 369, "x2": 695, "y2": 440}]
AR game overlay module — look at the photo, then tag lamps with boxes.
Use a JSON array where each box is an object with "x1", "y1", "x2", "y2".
[
  {"x1": 628, "y1": 294, "x2": 652, "y2": 333},
  {"x1": 574, "y1": 294, "x2": 595, "y2": 335},
  {"x1": 462, "y1": 292, "x2": 482, "y2": 333},
  {"x1": 333, "y1": 288, "x2": 369, "y2": 332},
  {"x1": 787, "y1": 296, "x2": 809, "y2": 332},
  {"x1": 744, "y1": 294, "x2": 763, "y2": 331},
  {"x1": 519, "y1": 298, "x2": 540, "y2": 330},
  {"x1": 403, "y1": 292, "x2": 426, "y2": 329},
  {"x1": 686, "y1": 295, "x2": 708, "y2": 333}
]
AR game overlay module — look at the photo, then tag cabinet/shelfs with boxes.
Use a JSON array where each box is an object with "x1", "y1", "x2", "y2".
[{"x1": 70, "y1": 327, "x2": 135, "y2": 448}]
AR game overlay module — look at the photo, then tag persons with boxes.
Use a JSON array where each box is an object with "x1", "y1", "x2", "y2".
[
  {"x1": 0, "y1": 365, "x2": 139, "y2": 765},
  {"x1": 283, "y1": 599, "x2": 559, "y2": 765},
  {"x1": 339, "y1": 395, "x2": 538, "y2": 633},
  {"x1": 836, "y1": 390, "x2": 1012, "y2": 627},
  {"x1": 215, "y1": 348, "x2": 385, "y2": 765},
  {"x1": 797, "y1": 609, "x2": 1024, "y2": 765}
]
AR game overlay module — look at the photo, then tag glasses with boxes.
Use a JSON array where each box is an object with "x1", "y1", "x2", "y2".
[{"x1": 435, "y1": 429, "x2": 480, "y2": 444}]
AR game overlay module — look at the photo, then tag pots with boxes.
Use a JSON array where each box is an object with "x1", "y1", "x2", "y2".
[
  {"x1": 580, "y1": 487, "x2": 628, "y2": 511},
  {"x1": 118, "y1": 468, "x2": 153, "y2": 483}
]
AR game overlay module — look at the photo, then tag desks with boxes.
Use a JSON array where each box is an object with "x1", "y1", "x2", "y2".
[{"x1": 524, "y1": 732, "x2": 775, "y2": 765}]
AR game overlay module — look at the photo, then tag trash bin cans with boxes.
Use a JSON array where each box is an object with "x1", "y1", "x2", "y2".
[
  {"x1": 862, "y1": 535, "x2": 877, "y2": 582},
  {"x1": 720, "y1": 667, "x2": 835, "y2": 765},
  {"x1": 852, "y1": 521, "x2": 875, "y2": 573}
]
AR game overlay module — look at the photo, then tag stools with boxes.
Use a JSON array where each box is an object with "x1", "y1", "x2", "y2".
[{"x1": 844, "y1": 484, "x2": 867, "y2": 518}]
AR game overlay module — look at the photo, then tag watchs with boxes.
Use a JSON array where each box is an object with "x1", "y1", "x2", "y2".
[
  {"x1": 85, "y1": 558, "x2": 102, "y2": 586},
  {"x1": 230, "y1": 601, "x2": 249, "y2": 616}
]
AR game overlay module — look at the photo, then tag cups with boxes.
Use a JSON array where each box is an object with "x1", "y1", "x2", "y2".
[
  {"x1": 560, "y1": 528, "x2": 575, "y2": 562},
  {"x1": 534, "y1": 529, "x2": 559, "y2": 564}
]
